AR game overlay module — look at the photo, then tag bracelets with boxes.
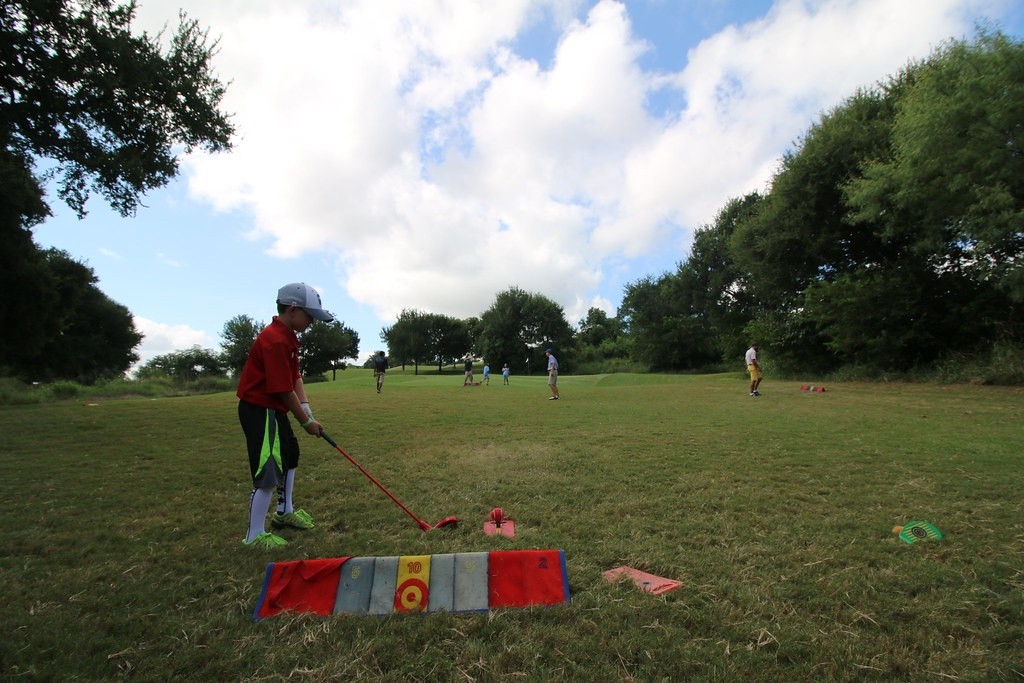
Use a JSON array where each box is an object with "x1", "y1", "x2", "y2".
[{"x1": 301, "y1": 418, "x2": 312, "y2": 426}]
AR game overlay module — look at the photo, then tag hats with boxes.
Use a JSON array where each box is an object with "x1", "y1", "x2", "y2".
[
  {"x1": 276, "y1": 282, "x2": 334, "y2": 322},
  {"x1": 544, "y1": 349, "x2": 552, "y2": 353}
]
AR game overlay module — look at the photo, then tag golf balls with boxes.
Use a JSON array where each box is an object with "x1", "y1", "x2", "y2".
[{"x1": 490, "y1": 508, "x2": 505, "y2": 522}]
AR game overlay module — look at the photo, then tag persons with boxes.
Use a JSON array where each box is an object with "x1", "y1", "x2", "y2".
[
  {"x1": 480, "y1": 362, "x2": 489, "y2": 385},
  {"x1": 745, "y1": 343, "x2": 763, "y2": 396},
  {"x1": 236, "y1": 283, "x2": 323, "y2": 550},
  {"x1": 374, "y1": 351, "x2": 389, "y2": 393},
  {"x1": 463, "y1": 353, "x2": 473, "y2": 386},
  {"x1": 502, "y1": 363, "x2": 510, "y2": 385},
  {"x1": 544, "y1": 349, "x2": 560, "y2": 400}
]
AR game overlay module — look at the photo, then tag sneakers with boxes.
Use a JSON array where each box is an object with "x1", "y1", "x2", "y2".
[
  {"x1": 242, "y1": 531, "x2": 288, "y2": 550},
  {"x1": 271, "y1": 509, "x2": 315, "y2": 529}
]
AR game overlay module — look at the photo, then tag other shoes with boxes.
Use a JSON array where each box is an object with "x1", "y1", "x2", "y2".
[
  {"x1": 750, "y1": 391, "x2": 761, "y2": 396},
  {"x1": 549, "y1": 396, "x2": 560, "y2": 400}
]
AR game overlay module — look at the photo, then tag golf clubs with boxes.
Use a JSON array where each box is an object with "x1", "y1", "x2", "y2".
[{"x1": 319, "y1": 430, "x2": 458, "y2": 532}]
